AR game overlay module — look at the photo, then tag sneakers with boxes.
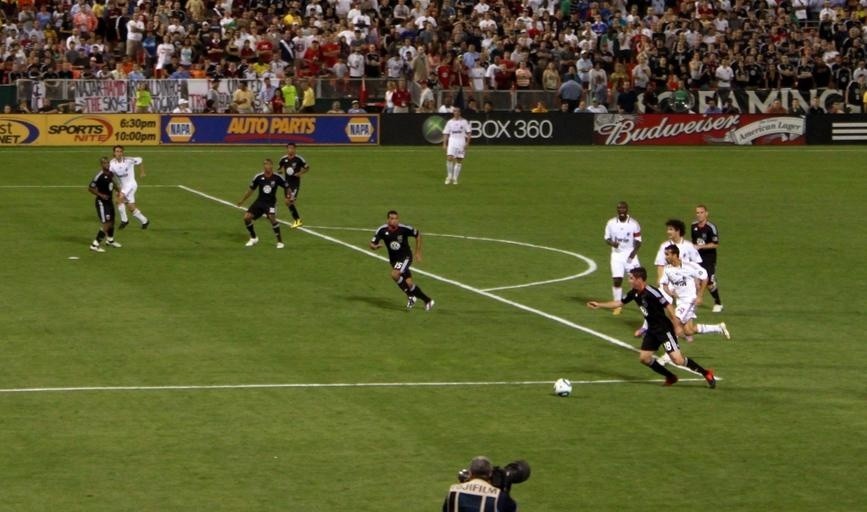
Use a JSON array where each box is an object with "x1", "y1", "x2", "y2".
[
  {"x1": 105, "y1": 240, "x2": 122, "y2": 248},
  {"x1": 119, "y1": 220, "x2": 128, "y2": 229},
  {"x1": 425, "y1": 299, "x2": 435, "y2": 311},
  {"x1": 712, "y1": 305, "x2": 723, "y2": 312},
  {"x1": 90, "y1": 244, "x2": 105, "y2": 252},
  {"x1": 291, "y1": 218, "x2": 302, "y2": 228},
  {"x1": 407, "y1": 296, "x2": 417, "y2": 308},
  {"x1": 612, "y1": 308, "x2": 622, "y2": 315},
  {"x1": 453, "y1": 176, "x2": 458, "y2": 185},
  {"x1": 445, "y1": 175, "x2": 452, "y2": 184},
  {"x1": 658, "y1": 353, "x2": 670, "y2": 366},
  {"x1": 634, "y1": 327, "x2": 645, "y2": 337},
  {"x1": 245, "y1": 237, "x2": 259, "y2": 247},
  {"x1": 705, "y1": 369, "x2": 716, "y2": 388},
  {"x1": 277, "y1": 242, "x2": 284, "y2": 249},
  {"x1": 665, "y1": 375, "x2": 677, "y2": 385},
  {"x1": 721, "y1": 322, "x2": 730, "y2": 339},
  {"x1": 142, "y1": 220, "x2": 149, "y2": 230}
]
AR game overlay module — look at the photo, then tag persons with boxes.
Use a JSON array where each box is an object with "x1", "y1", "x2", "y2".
[
  {"x1": 441, "y1": 106, "x2": 472, "y2": 186},
  {"x1": 1, "y1": 1, "x2": 867, "y2": 115},
  {"x1": 369, "y1": 211, "x2": 435, "y2": 311},
  {"x1": 109, "y1": 145, "x2": 150, "y2": 230},
  {"x1": 585, "y1": 201, "x2": 731, "y2": 390},
  {"x1": 441, "y1": 456, "x2": 516, "y2": 512},
  {"x1": 88, "y1": 155, "x2": 123, "y2": 253},
  {"x1": 276, "y1": 143, "x2": 311, "y2": 229},
  {"x1": 236, "y1": 159, "x2": 292, "y2": 249}
]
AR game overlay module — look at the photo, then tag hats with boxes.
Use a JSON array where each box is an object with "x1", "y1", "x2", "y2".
[{"x1": 178, "y1": 98, "x2": 189, "y2": 105}]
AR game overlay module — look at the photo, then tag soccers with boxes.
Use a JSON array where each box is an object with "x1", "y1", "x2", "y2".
[{"x1": 553, "y1": 378, "x2": 572, "y2": 397}]
